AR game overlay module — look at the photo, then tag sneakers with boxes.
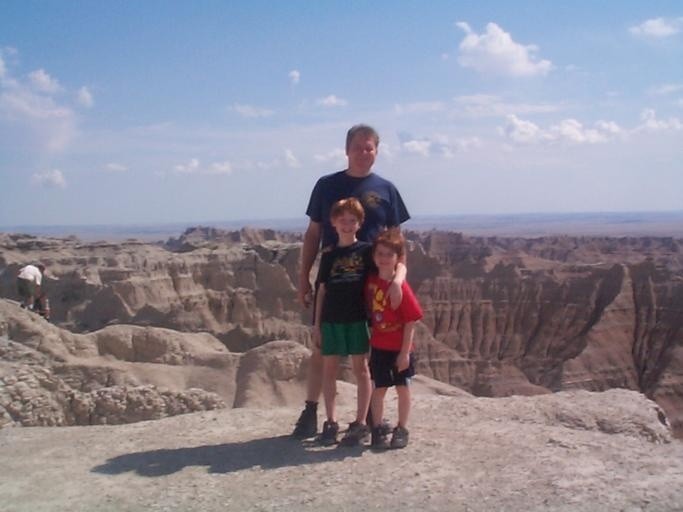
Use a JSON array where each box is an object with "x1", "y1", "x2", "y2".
[
  {"x1": 342, "y1": 421, "x2": 369, "y2": 445},
  {"x1": 390, "y1": 426, "x2": 408, "y2": 449},
  {"x1": 323, "y1": 421, "x2": 339, "y2": 445},
  {"x1": 371, "y1": 427, "x2": 387, "y2": 449},
  {"x1": 295, "y1": 411, "x2": 317, "y2": 437}
]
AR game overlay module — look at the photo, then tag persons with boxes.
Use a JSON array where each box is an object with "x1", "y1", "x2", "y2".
[
  {"x1": 308, "y1": 194, "x2": 406, "y2": 447},
  {"x1": 286, "y1": 123, "x2": 414, "y2": 441},
  {"x1": 36, "y1": 289, "x2": 49, "y2": 318},
  {"x1": 361, "y1": 226, "x2": 422, "y2": 453},
  {"x1": 16, "y1": 261, "x2": 44, "y2": 311}
]
